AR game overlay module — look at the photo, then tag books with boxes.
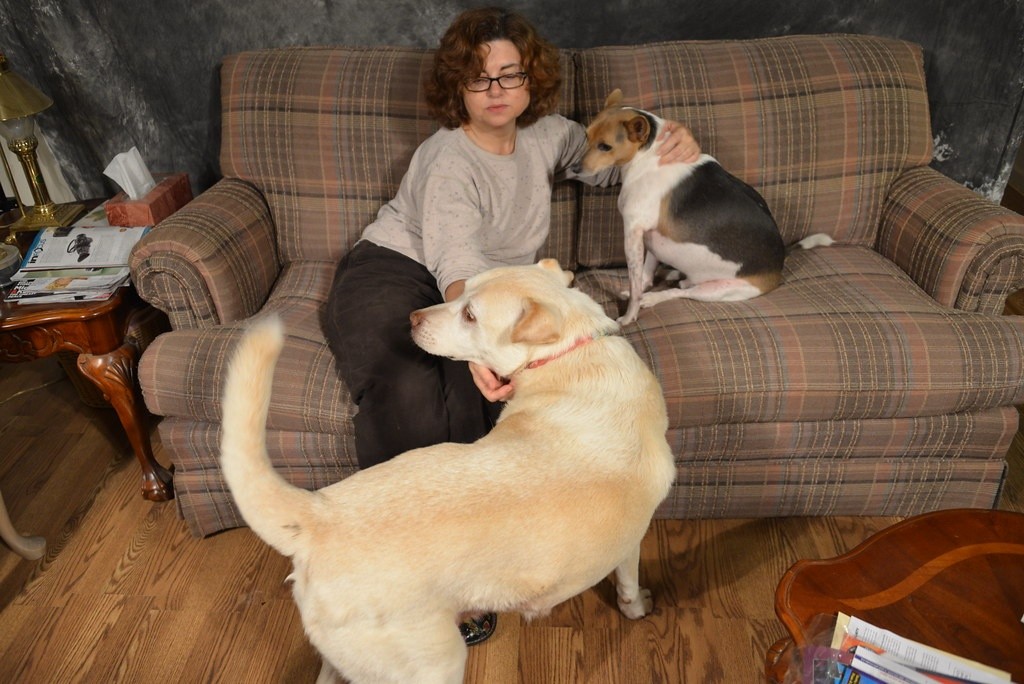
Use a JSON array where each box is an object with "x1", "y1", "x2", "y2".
[
  {"x1": 4, "y1": 198, "x2": 156, "y2": 305},
  {"x1": 828, "y1": 612, "x2": 1016, "y2": 684}
]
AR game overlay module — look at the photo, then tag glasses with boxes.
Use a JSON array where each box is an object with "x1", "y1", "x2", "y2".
[{"x1": 463, "y1": 72, "x2": 528, "y2": 92}]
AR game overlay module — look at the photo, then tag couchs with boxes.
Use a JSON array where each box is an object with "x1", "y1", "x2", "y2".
[{"x1": 128, "y1": 34, "x2": 1024, "y2": 539}]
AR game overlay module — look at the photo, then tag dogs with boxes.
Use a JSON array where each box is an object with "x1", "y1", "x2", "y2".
[
  {"x1": 217, "y1": 257, "x2": 675, "y2": 684},
  {"x1": 570, "y1": 88, "x2": 786, "y2": 326}
]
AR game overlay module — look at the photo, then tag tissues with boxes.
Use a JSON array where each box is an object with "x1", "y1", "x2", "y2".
[{"x1": 102, "y1": 145, "x2": 191, "y2": 226}]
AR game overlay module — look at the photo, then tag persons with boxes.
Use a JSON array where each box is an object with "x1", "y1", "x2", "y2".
[{"x1": 322, "y1": 1, "x2": 703, "y2": 645}]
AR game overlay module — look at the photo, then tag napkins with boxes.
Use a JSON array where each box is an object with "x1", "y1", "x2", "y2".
[{"x1": 101, "y1": 146, "x2": 157, "y2": 201}]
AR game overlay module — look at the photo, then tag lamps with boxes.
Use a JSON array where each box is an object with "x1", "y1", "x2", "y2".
[{"x1": 0, "y1": 49, "x2": 86, "y2": 233}]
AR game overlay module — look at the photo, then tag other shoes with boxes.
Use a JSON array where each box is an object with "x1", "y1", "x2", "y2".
[{"x1": 458, "y1": 612, "x2": 497, "y2": 646}]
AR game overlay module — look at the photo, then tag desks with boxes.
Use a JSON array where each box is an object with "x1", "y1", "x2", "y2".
[
  {"x1": 0, "y1": 199, "x2": 174, "y2": 504},
  {"x1": 766, "y1": 509, "x2": 1024, "y2": 684}
]
